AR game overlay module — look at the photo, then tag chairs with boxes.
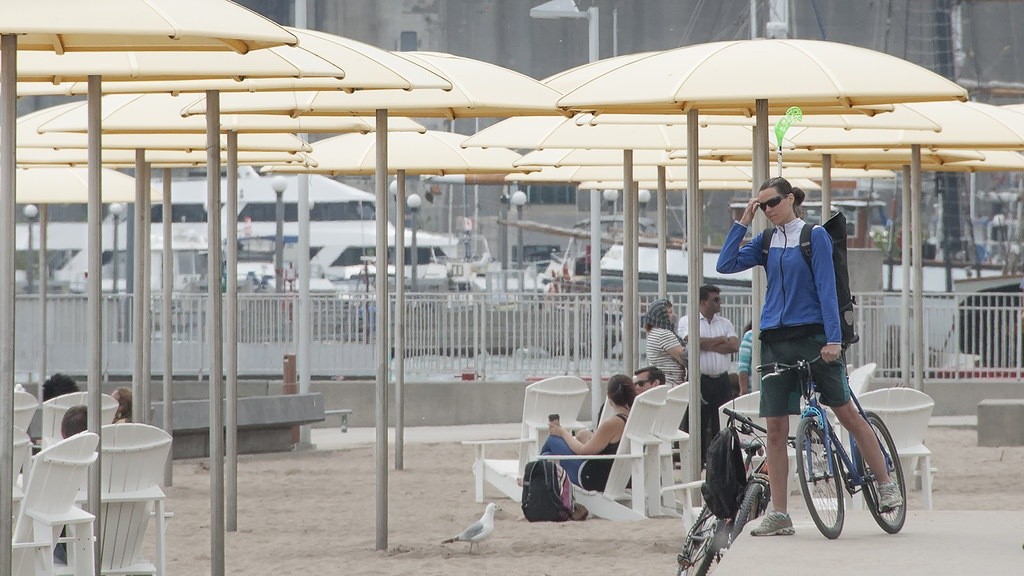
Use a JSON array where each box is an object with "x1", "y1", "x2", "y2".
[
  {"x1": 460, "y1": 364, "x2": 934, "y2": 521},
  {"x1": 9, "y1": 389, "x2": 173, "y2": 576}
]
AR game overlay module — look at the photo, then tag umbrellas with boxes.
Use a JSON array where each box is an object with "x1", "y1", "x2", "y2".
[{"x1": 0, "y1": 0, "x2": 1024, "y2": 576}]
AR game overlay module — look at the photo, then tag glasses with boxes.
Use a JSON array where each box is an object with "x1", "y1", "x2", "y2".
[
  {"x1": 760, "y1": 194, "x2": 788, "y2": 211},
  {"x1": 704, "y1": 297, "x2": 720, "y2": 302},
  {"x1": 634, "y1": 377, "x2": 657, "y2": 387}
]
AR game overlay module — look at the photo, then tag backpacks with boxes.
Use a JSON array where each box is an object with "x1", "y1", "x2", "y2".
[
  {"x1": 701, "y1": 415, "x2": 746, "y2": 518},
  {"x1": 521, "y1": 460, "x2": 574, "y2": 521},
  {"x1": 762, "y1": 212, "x2": 859, "y2": 343}
]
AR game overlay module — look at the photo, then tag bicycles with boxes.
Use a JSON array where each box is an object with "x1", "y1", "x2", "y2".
[
  {"x1": 752, "y1": 350, "x2": 910, "y2": 541},
  {"x1": 671, "y1": 406, "x2": 823, "y2": 576}
]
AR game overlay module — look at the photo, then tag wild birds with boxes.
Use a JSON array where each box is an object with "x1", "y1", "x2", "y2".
[{"x1": 440, "y1": 502, "x2": 503, "y2": 555}]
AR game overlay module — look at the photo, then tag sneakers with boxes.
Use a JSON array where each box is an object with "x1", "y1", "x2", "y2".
[
  {"x1": 751, "y1": 511, "x2": 794, "y2": 536},
  {"x1": 877, "y1": 481, "x2": 902, "y2": 511}
]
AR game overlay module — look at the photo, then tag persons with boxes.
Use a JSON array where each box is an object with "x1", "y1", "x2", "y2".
[
  {"x1": 61, "y1": 405, "x2": 87, "y2": 439},
  {"x1": 42, "y1": 373, "x2": 80, "y2": 402},
  {"x1": 642, "y1": 298, "x2": 688, "y2": 384},
  {"x1": 111, "y1": 387, "x2": 132, "y2": 423},
  {"x1": 632, "y1": 366, "x2": 666, "y2": 395},
  {"x1": 738, "y1": 322, "x2": 752, "y2": 396},
  {"x1": 716, "y1": 177, "x2": 903, "y2": 537},
  {"x1": 677, "y1": 285, "x2": 739, "y2": 467},
  {"x1": 516, "y1": 374, "x2": 637, "y2": 491}
]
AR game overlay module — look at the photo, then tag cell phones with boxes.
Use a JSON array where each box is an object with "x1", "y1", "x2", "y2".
[{"x1": 549, "y1": 414, "x2": 560, "y2": 426}]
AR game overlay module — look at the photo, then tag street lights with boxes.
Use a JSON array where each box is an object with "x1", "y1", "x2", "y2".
[
  {"x1": 405, "y1": 192, "x2": 422, "y2": 296},
  {"x1": 107, "y1": 200, "x2": 124, "y2": 296},
  {"x1": 270, "y1": 173, "x2": 289, "y2": 298},
  {"x1": 513, "y1": 190, "x2": 530, "y2": 297},
  {"x1": 602, "y1": 187, "x2": 621, "y2": 233},
  {"x1": 21, "y1": 205, "x2": 38, "y2": 298},
  {"x1": 637, "y1": 188, "x2": 653, "y2": 221}
]
